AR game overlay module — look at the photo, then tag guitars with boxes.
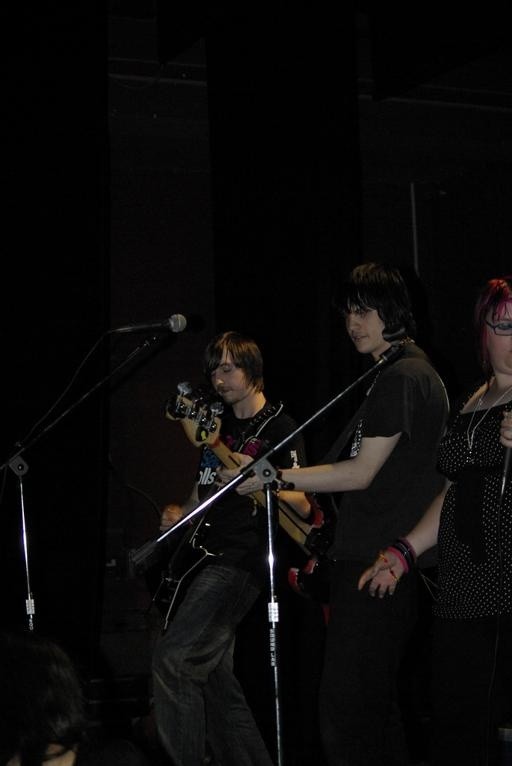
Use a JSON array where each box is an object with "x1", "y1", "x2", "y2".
[
  {"x1": 165, "y1": 382, "x2": 337, "y2": 624},
  {"x1": 153, "y1": 401, "x2": 284, "y2": 630}
]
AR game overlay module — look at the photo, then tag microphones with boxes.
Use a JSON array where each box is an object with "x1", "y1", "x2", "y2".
[
  {"x1": 500, "y1": 410, "x2": 512, "y2": 496},
  {"x1": 110, "y1": 313, "x2": 187, "y2": 333}
]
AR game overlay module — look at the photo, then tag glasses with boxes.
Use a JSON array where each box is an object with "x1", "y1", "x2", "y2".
[{"x1": 483, "y1": 317, "x2": 512, "y2": 337}]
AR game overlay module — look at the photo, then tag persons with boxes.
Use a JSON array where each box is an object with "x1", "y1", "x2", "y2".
[{"x1": 153, "y1": 262, "x2": 512, "y2": 766}]
